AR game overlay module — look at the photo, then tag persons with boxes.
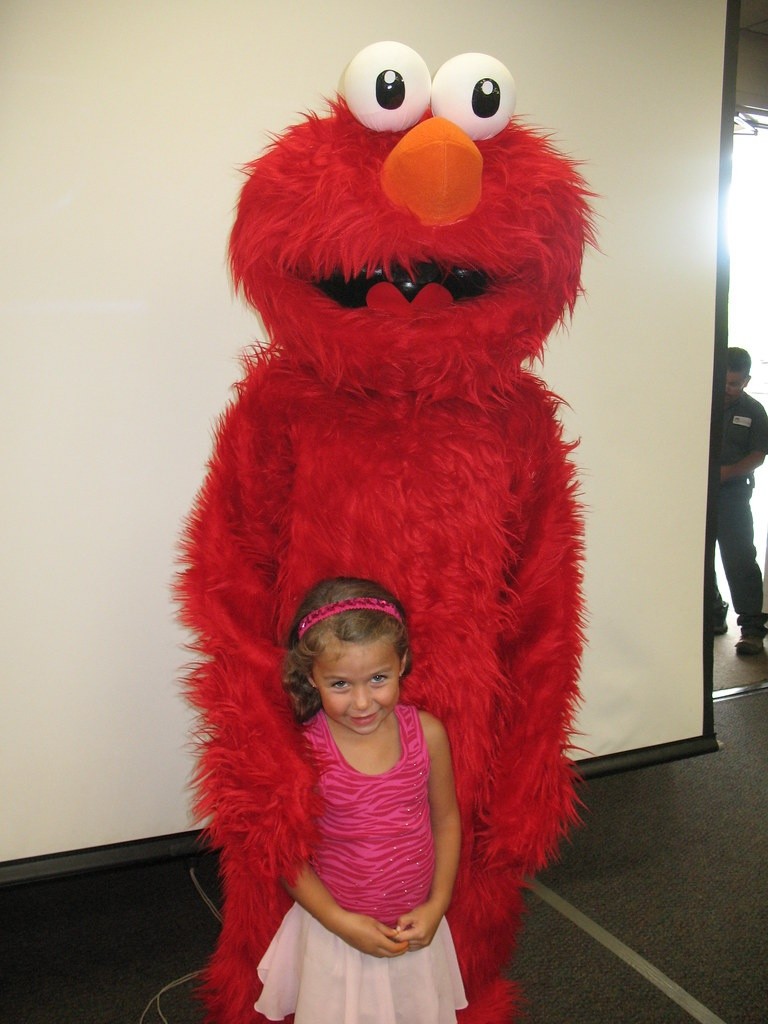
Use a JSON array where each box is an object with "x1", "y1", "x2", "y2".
[
  {"x1": 713, "y1": 347, "x2": 768, "y2": 652},
  {"x1": 254, "y1": 576, "x2": 469, "y2": 1024}
]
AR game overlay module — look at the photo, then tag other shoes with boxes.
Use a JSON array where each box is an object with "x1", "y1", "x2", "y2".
[
  {"x1": 713, "y1": 601, "x2": 728, "y2": 633},
  {"x1": 736, "y1": 626, "x2": 768, "y2": 652}
]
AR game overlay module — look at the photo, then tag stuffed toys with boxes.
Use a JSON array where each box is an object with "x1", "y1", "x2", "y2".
[{"x1": 172, "y1": 41, "x2": 591, "y2": 1024}]
